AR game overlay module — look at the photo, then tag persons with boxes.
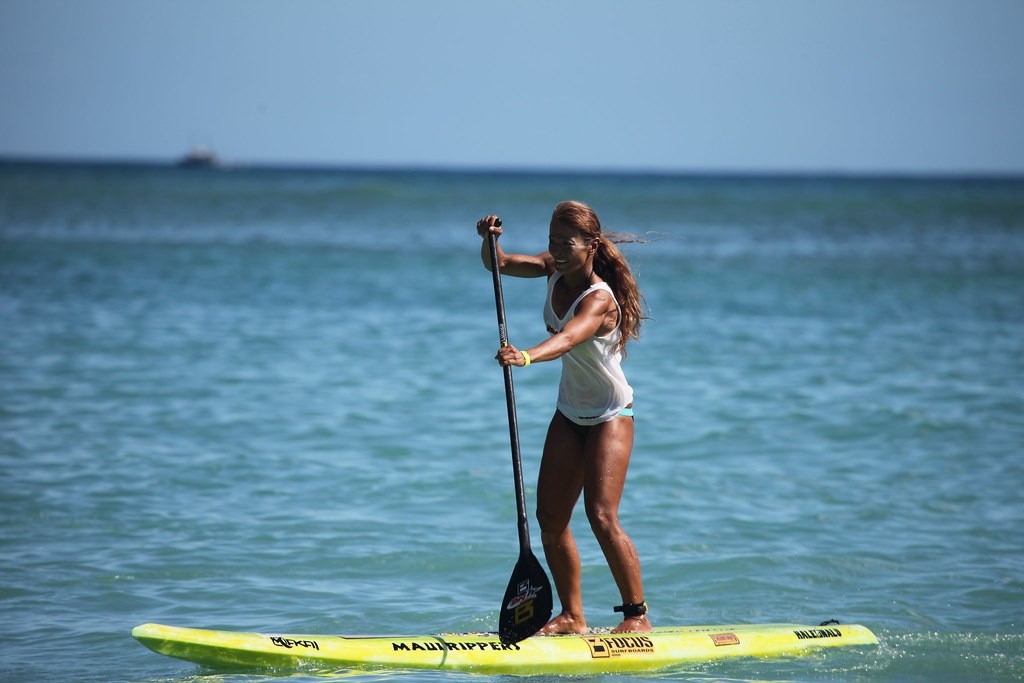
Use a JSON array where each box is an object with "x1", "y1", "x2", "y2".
[{"x1": 477, "y1": 200, "x2": 652, "y2": 636}]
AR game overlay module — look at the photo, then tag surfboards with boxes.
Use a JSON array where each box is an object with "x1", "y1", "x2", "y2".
[{"x1": 131, "y1": 624, "x2": 882, "y2": 675}]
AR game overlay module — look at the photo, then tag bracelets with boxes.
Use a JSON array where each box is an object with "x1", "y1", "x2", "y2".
[{"x1": 519, "y1": 351, "x2": 530, "y2": 367}]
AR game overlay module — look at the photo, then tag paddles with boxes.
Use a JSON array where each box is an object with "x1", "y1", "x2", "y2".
[{"x1": 486, "y1": 220, "x2": 554, "y2": 648}]
